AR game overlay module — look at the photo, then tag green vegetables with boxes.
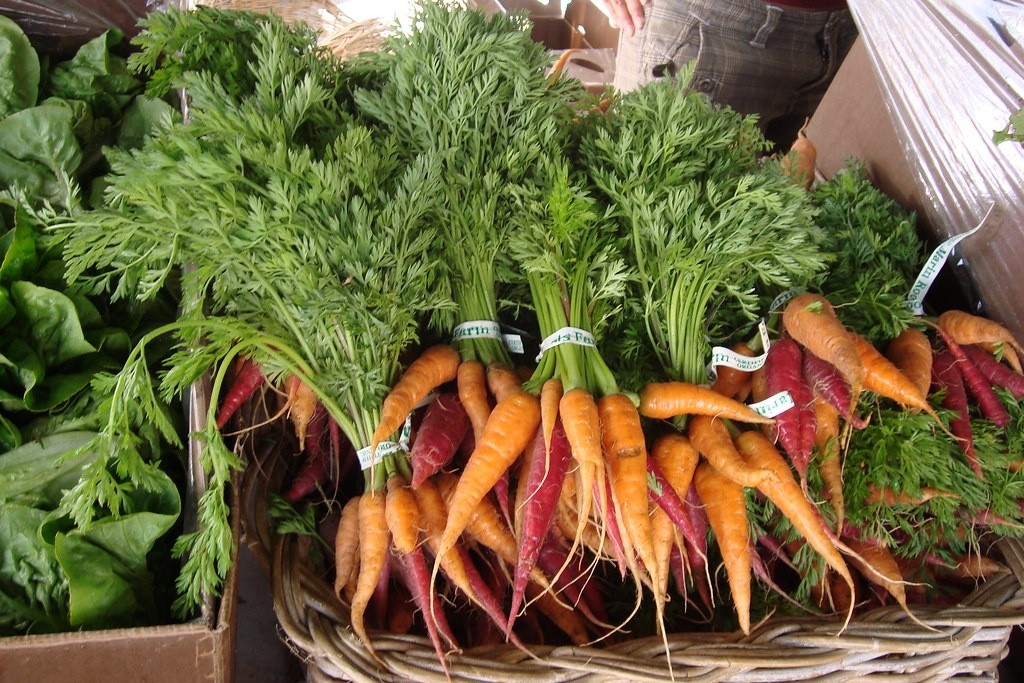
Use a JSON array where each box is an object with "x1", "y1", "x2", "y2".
[{"x1": 0, "y1": 14, "x2": 189, "y2": 639}]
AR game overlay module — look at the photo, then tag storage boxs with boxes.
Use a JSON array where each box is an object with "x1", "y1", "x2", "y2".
[
  {"x1": 803, "y1": 0, "x2": 1024, "y2": 342},
  {"x1": 0, "y1": 374, "x2": 245, "y2": 683},
  {"x1": 468, "y1": 0, "x2": 620, "y2": 99}
]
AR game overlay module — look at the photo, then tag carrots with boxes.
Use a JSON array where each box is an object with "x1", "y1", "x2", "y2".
[
  {"x1": 778, "y1": 115, "x2": 817, "y2": 191},
  {"x1": 211, "y1": 294, "x2": 1024, "y2": 683}
]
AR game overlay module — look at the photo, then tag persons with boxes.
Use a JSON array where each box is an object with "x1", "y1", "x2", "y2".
[{"x1": 604, "y1": 0, "x2": 859, "y2": 160}]
